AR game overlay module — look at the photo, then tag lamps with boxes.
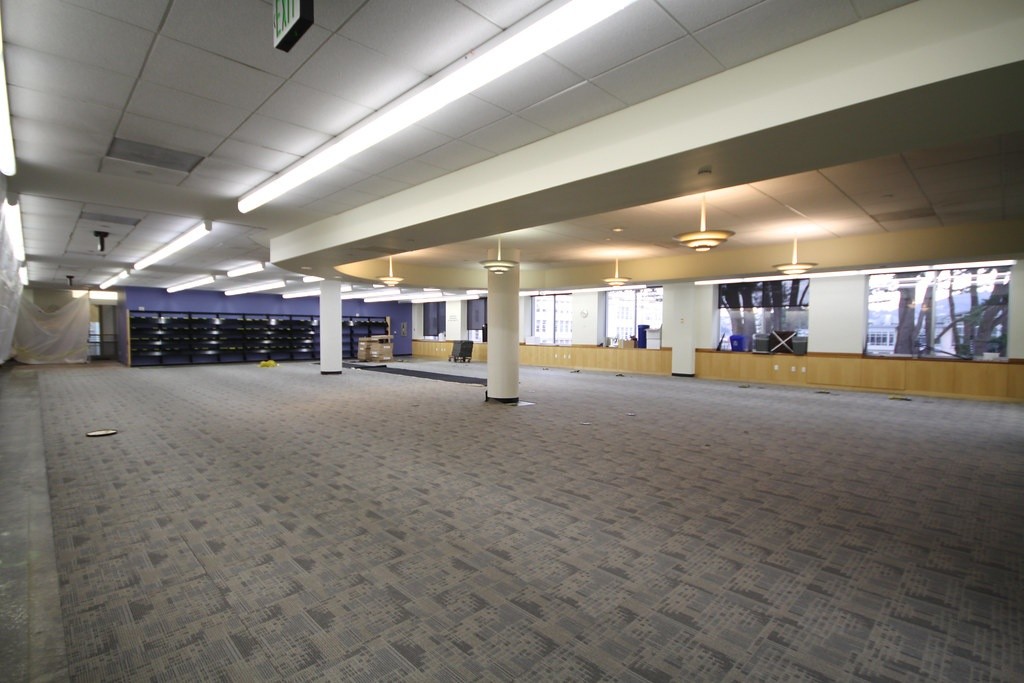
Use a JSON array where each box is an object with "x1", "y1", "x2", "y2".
[
  {"x1": 675, "y1": 194, "x2": 736, "y2": 253},
  {"x1": 4, "y1": 191, "x2": 27, "y2": 262},
  {"x1": 133, "y1": 217, "x2": 212, "y2": 271},
  {"x1": 237, "y1": 0, "x2": 636, "y2": 212},
  {"x1": 600, "y1": 257, "x2": 632, "y2": 288},
  {"x1": 478, "y1": 236, "x2": 521, "y2": 275},
  {"x1": 375, "y1": 255, "x2": 407, "y2": 287},
  {"x1": 771, "y1": 236, "x2": 818, "y2": 276}
]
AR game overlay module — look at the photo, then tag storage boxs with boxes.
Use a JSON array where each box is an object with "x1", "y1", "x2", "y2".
[
  {"x1": 623, "y1": 340, "x2": 637, "y2": 348},
  {"x1": 356, "y1": 335, "x2": 395, "y2": 363}
]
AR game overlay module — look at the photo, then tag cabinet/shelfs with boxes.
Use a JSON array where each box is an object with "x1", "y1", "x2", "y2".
[{"x1": 124, "y1": 309, "x2": 391, "y2": 368}]
